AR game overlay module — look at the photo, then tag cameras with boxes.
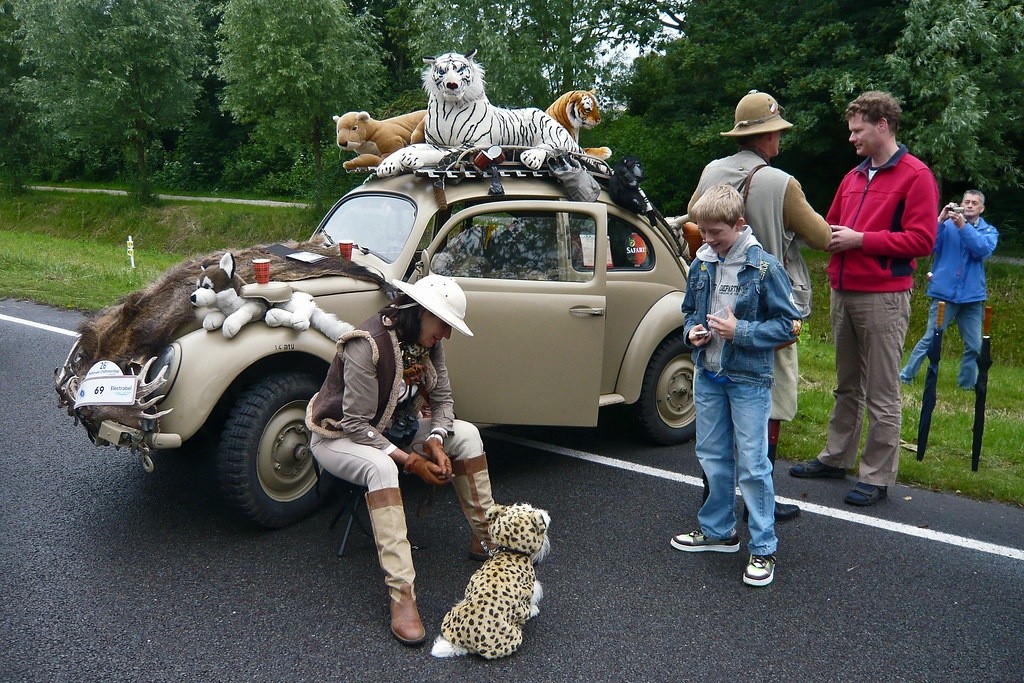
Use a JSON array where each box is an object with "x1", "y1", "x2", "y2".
[{"x1": 949, "y1": 205, "x2": 964, "y2": 213}]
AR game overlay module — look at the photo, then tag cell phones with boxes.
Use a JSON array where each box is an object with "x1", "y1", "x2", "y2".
[{"x1": 695, "y1": 330, "x2": 708, "y2": 338}]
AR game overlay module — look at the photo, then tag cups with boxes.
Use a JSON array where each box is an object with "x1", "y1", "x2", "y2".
[
  {"x1": 711, "y1": 307, "x2": 729, "y2": 325},
  {"x1": 488, "y1": 146, "x2": 505, "y2": 164},
  {"x1": 252, "y1": 259, "x2": 272, "y2": 285},
  {"x1": 339, "y1": 241, "x2": 353, "y2": 261},
  {"x1": 474, "y1": 150, "x2": 493, "y2": 170}
]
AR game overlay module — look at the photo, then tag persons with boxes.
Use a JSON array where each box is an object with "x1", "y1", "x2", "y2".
[
  {"x1": 899, "y1": 190, "x2": 999, "y2": 390},
  {"x1": 688, "y1": 91, "x2": 833, "y2": 521},
  {"x1": 788, "y1": 92, "x2": 938, "y2": 505},
  {"x1": 304, "y1": 275, "x2": 498, "y2": 646},
  {"x1": 670, "y1": 184, "x2": 802, "y2": 587}
]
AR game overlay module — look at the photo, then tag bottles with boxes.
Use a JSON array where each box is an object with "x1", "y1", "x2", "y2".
[{"x1": 926, "y1": 272, "x2": 934, "y2": 282}]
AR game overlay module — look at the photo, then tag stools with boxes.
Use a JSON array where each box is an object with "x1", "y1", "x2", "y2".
[{"x1": 330, "y1": 475, "x2": 369, "y2": 556}]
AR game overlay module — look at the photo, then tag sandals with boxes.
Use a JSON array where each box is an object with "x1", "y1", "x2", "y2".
[
  {"x1": 844, "y1": 480, "x2": 888, "y2": 506},
  {"x1": 790, "y1": 457, "x2": 848, "y2": 478}
]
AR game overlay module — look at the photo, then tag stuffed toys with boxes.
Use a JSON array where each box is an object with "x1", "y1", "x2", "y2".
[
  {"x1": 545, "y1": 89, "x2": 612, "y2": 160},
  {"x1": 609, "y1": 155, "x2": 647, "y2": 216},
  {"x1": 332, "y1": 109, "x2": 427, "y2": 173},
  {"x1": 189, "y1": 252, "x2": 355, "y2": 342},
  {"x1": 377, "y1": 48, "x2": 657, "y2": 227},
  {"x1": 433, "y1": 503, "x2": 553, "y2": 658}
]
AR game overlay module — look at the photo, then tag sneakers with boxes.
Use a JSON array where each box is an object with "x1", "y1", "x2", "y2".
[
  {"x1": 742, "y1": 552, "x2": 778, "y2": 587},
  {"x1": 670, "y1": 529, "x2": 741, "y2": 552}
]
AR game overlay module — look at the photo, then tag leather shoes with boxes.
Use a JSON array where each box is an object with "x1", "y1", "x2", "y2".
[{"x1": 741, "y1": 498, "x2": 801, "y2": 522}]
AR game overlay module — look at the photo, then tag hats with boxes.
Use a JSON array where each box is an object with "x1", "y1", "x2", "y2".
[
  {"x1": 392, "y1": 274, "x2": 475, "y2": 336},
  {"x1": 719, "y1": 89, "x2": 794, "y2": 137}
]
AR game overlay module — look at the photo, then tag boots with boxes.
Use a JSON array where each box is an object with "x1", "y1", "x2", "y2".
[
  {"x1": 449, "y1": 451, "x2": 496, "y2": 558},
  {"x1": 364, "y1": 488, "x2": 427, "y2": 645}
]
